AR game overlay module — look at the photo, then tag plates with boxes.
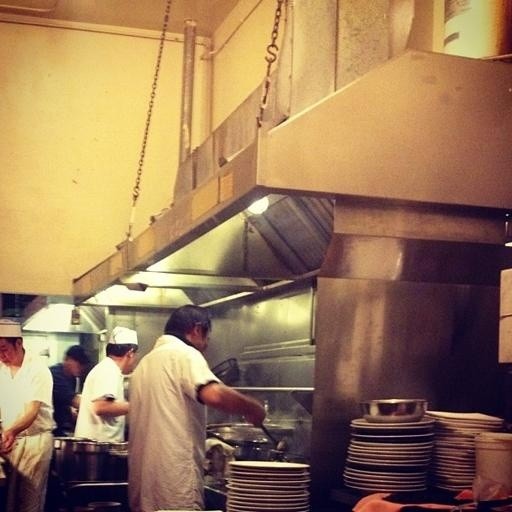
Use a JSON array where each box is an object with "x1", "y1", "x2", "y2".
[
  {"x1": 342, "y1": 410, "x2": 505, "y2": 497},
  {"x1": 225, "y1": 461, "x2": 312, "y2": 511}
]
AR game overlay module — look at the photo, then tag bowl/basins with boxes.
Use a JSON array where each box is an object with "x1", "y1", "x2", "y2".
[{"x1": 358, "y1": 398, "x2": 428, "y2": 422}]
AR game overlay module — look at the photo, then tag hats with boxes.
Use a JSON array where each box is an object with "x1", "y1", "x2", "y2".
[
  {"x1": 108, "y1": 326, "x2": 138, "y2": 345},
  {"x1": 0, "y1": 319, "x2": 22, "y2": 338},
  {"x1": 67, "y1": 345, "x2": 92, "y2": 364}
]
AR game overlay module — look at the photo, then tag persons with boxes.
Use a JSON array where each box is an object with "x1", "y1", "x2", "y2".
[
  {"x1": 49, "y1": 345, "x2": 98, "y2": 437},
  {"x1": 0, "y1": 318, "x2": 56, "y2": 511},
  {"x1": 126, "y1": 304, "x2": 268, "y2": 511},
  {"x1": 73, "y1": 326, "x2": 139, "y2": 442}
]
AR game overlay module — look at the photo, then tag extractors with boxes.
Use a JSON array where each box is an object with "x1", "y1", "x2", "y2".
[{"x1": 70, "y1": 145, "x2": 334, "y2": 316}]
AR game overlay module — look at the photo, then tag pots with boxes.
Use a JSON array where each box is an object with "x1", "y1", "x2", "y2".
[
  {"x1": 49, "y1": 439, "x2": 128, "y2": 483},
  {"x1": 207, "y1": 431, "x2": 276, "y2": 461}
]
